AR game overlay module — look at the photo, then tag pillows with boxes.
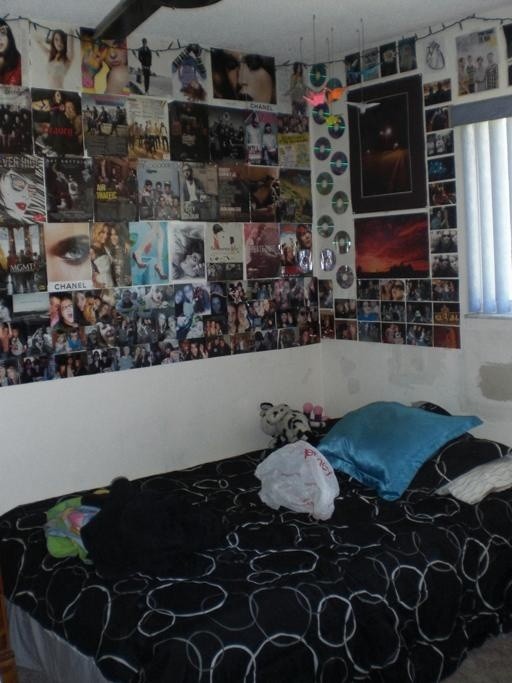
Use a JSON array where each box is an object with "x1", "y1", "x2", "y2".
[{"x1": 317, "y1": 400, "x2": 512, "y2": 506}]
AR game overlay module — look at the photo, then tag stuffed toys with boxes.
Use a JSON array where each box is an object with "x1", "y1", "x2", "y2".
[{"x1": 257, "y1": 399, "x2": 330, "y2": 449}]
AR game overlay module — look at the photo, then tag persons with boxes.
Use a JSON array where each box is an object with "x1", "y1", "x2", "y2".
[{"x1": 1, "y1": 12, "x2": 510, "y2": 393}]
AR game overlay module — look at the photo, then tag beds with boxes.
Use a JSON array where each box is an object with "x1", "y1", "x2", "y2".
[{"x1": 1, "y1": 447, "x2": 512, "y2": 683}]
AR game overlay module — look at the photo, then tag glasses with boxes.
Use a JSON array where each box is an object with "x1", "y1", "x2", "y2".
[{"x1": 5, "y1": 174, "x2": 37, "y2": 195}]
